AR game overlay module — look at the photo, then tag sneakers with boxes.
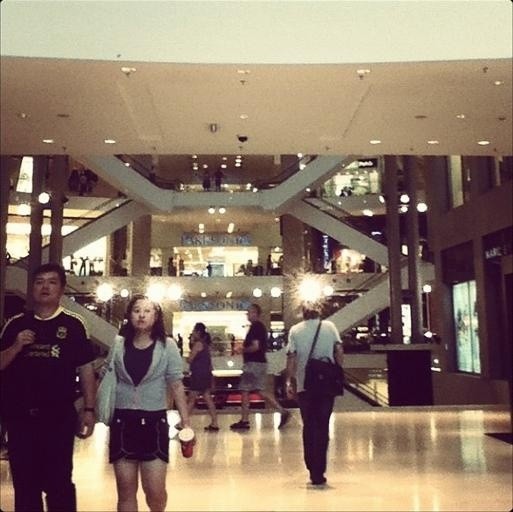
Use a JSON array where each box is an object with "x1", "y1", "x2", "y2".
[{"x1": 310, "y1": 471, "x2": 326, "y2": 485}]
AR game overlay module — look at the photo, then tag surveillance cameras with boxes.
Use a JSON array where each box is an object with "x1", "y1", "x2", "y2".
[{"x1": 238, "y1": 134, "x2": 248, "y2": 142}]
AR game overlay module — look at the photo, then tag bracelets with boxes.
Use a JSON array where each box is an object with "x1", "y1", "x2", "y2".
[
  {"x1": 84, "y1": 406, "x2": 96, "y2": 414},
  {"x1": 285, "y1": 380, "x2": 292, "y2": 387},
  {"x1": 180, "y1": 417, "x2": 190, "y2": 424}
]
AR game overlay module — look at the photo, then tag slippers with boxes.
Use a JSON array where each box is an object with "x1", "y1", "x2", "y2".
[
  {"x1": 175, "y1": 424, "x2": 183, "y2": 431},
  {"x1": 204, "y1": 424, "x2": 219, "y2": 431}
]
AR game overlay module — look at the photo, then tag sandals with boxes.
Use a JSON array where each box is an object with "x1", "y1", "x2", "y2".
[
  {"x1": 230, "y1": 420, "x2": 251, "y2": 429},
  {"x1": 278, "y1": 413, "x2": 292, "y2": 429}
]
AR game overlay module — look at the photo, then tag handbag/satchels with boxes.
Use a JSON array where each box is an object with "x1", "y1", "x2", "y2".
[
  {"x1": 210, "y1": 376, "x2": 216, "y2": 389},
  {"x1": 304, "y1": 359, "x2": 344, "y2": 396},
  {"x1": 94, "y1": 358, "x2": 117, "y2": 425}
]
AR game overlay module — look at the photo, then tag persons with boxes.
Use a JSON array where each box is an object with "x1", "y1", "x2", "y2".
[
  {"x1": 66, "y1": 163, "x2": 98, "y2": 197},
  {"x1": 201, "y1": 169, "x2": 211, "y2": 191},
  {"x1": 211, "y1": 167, "x2": 225, "y2": 192},
  {"x1": 206, "y1": 264, "x2": 212, "y2": 277},
  {"x1": 339, "y1": 185, "x2": 358, "y2": 197},
  {"x1": 282, "y1": 294, "x2": 344, "y2": 487},
  {"x1": 0, "y1": 261, "x2": 97, "y2": 512},
  {"x1": 246, "y1": 259, "x2": 256, "y2": 275},
  {"x1": 265, "y1": 254, "x2": 273, "y2": 275},
  {"x1": 93, "y1": 293, "x2": 198, "y2": 512},
  {"x1": 163, "y1": 329, "x2": 288, "y2": 359},
  {"x1": 148, "y1": 164, "x2": 157, "y2": 186},
  {"x1": 228, "y1": 303, "x2": 292, "y2": 431},
  {"x1": 173, "y1": 322, "x2": 221, "y2": 431}
]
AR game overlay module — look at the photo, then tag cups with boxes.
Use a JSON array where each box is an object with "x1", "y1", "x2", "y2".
[{"x1": 178, "y1": 428, "x2": 195, "y2": 458}]
